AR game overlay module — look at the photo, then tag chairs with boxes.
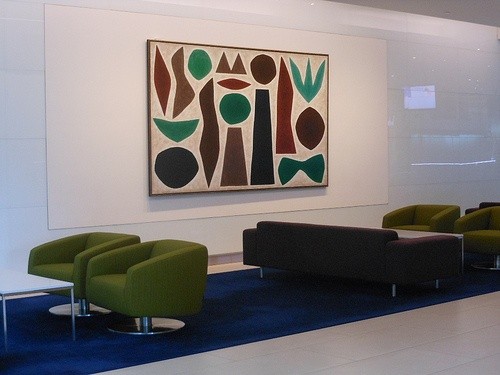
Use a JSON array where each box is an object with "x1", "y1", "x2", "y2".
[
  {"x1": 454, "y1": 205, "x2": 500, "y2": 270},
  {"x1": 382, "y1": 204, "x2": 461, "y2": 233},
  {"x1": 86, "y1": 239, "x2": 208, "y2": 334},
  {"x1": 27, "y1": 232, "x2": 141, "y2": 317}
]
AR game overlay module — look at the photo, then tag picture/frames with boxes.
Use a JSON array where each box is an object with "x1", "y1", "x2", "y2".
[{"x1": 146, "y1": 39, "x2": 329, "y2": 197}]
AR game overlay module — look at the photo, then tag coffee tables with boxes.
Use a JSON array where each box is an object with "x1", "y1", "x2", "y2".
[
  {"x1": 0, "y1": 271, "x2": 76, "y2": 346},
  {"x1": 366, "y1": 225, "x2": 464, "y2": 288}
]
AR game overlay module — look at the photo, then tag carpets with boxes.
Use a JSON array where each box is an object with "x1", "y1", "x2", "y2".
[{"x1": 0, "y1": 255, "x2": 499, "y2": 375}]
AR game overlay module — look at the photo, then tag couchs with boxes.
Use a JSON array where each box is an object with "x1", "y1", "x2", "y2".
[
  {"x1": 243, "y1": 221, "x2": 461, "y2": 295},
  {"x1": 465, "y1": 201, "x2": 500, "y2": 215}
]
